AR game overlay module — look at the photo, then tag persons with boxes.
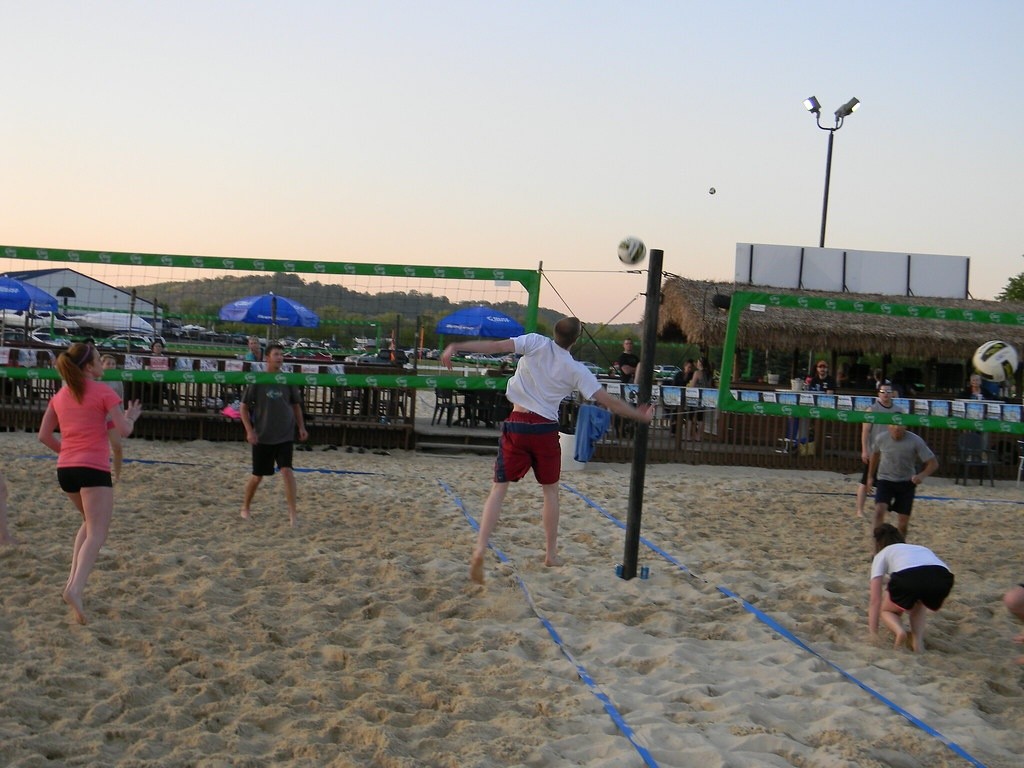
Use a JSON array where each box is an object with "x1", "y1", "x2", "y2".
[
  {"x1": 957, "y1": 373, "x2": 992, "y2": 464},
  {"x1": 441, "y1": 318, "x2": 654, "y2": 584},
  {"x1": 1006, "y1": 582, "x2": 1024, "y2": 668},
  {"x1": 857, "y1": 378, "x2": 908, "y2": 518},
  {"x1": 868, "y1": 412, "x2": 938, "y2": 562},
  {"x1": 239, "y1": 345, "x2": 309, "y2": 526},
  {"x1": 686, "y1": 355, "x2": 713, "y2": 434},
  {"x1": 36, "y1": 343, "x2": 142, "y2": 626},
  {"x1": 869, "y1": 523, "x2": 954, "y2": 653},
  {"x1": 97, "y1": 353, "x2": 125, "y2": 483},
  {"x1": 150, "y1": 341, "x2": 165, "y2": 357},
  {"x1": 245, "y1": 335, "x2": 264, "y2": 361},
  {"x1": 671, "y1": 359, "x2": 694, "y2": 439},
  {"x1": 619, "y1": 338, "x2": 639, "y2": 385},
  {"x1": 874, "y1": 368, "x2": 892, "y2": 390}
]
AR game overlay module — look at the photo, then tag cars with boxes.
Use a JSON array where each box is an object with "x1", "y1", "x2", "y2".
[
  {"x1": 465, "y1": 352, "x2": 501, "y2": 362},
  {"x1": 280, "y1": 338, "x2": 338, "y2": 349},
  {"x1": 0, "y1": 308, "x2": 79, "y2": 332},
  {"x1": 0, "y1": 332, "x2": 67, "y2": 368},
  {"x1": 404, "y1": 348, "x2": 459, "y2": 361},
  {"x1": 164, "y1": 322, "x2": 278, "y2": 348},
  {"x1": 95, "y1": 334, "x2": 164, "y2": 363},
  {"x1": 579, "y1": 361, "x2": 602, "y2": 372},
  {"x1": 345, "y1": 352, "x2": 390, "y2": 364}
]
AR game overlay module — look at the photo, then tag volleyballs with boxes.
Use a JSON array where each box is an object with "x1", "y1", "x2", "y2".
[
  {"x1": 618, "y1": 236, "x2": 647, "y2": 265},
  {"x1": 971, "y1": 340, "x2": 1019, "y2": 383}
]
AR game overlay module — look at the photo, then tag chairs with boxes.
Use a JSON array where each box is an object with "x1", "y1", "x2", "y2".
[
  {"x1": 954, "y1": 433, "x2": 997, "y2": 487},
  {"x1": 1015, "y1": 440, "x2": 1024, "y2": 483},
  {"x1": 431, "y1": 370, "x2": 513, "y2": 429}
]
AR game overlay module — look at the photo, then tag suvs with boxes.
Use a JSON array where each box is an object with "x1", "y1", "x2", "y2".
[
  {"x1": 283, "y1": 346, "x2": 333, "y2": 362},
  {"x1": 654, "y1": 365, "x2": 682, "y2": 377}
]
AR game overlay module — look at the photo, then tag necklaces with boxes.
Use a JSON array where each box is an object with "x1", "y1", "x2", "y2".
[{"x1": 808, "y1": 360, "x2": 835, "y2": 394}]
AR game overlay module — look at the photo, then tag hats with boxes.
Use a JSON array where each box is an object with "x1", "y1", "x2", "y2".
[
  {"x1": 364, "y1": 339, "x2": 376, "y2": 348},
  {"x1": 817, "y1": 360, "x2": 829, "y2": 368}
]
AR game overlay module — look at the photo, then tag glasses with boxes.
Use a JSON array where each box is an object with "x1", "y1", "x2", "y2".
[
  {"x1": 879, "y1": 389, "x2": 893, "y2": 393},
  {"x1": 576, "y1": 324, "x2": 584, "y2": 339},
  {"x1": 625, "y1": 343, "x2": 633, "y2": 345}
]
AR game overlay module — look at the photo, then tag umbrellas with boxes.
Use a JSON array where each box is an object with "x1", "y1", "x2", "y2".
[
  {"x1": 0, "y1": 273, "x2": 58, "y2": 347},
  {"x1": 434, "y1": 306, "x2": 524, "y2": 341},
  {"x1": 218, "y1": 291, "x2": 320, "y2": 338}
]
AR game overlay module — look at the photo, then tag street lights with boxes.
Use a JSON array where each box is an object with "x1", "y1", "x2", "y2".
[{"x1": 803, "y1": 96, "x2": 860, "y2": 248}]
